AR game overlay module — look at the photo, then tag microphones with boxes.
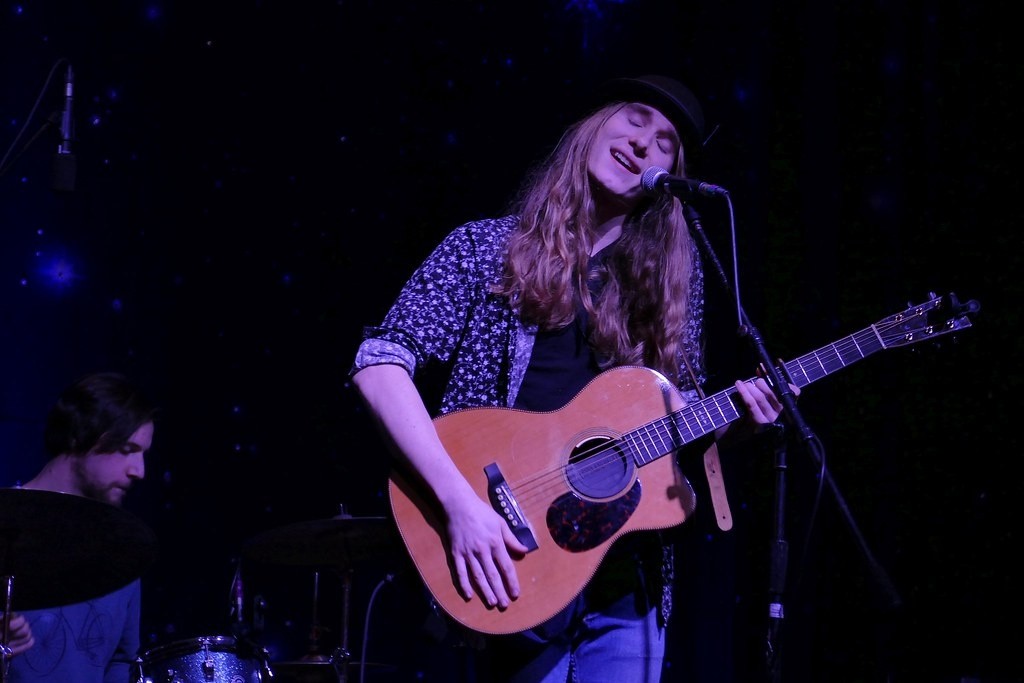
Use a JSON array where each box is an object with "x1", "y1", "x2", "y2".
[
  {"x1": 232, "y1": 558, "x2": 244, "y2": 621},
  {"x1": 61, "y1": 65, "x2": 75, "y2": 154},
  {"x1": 640, "y1": 166, "x2": 731, "y2": 198}
]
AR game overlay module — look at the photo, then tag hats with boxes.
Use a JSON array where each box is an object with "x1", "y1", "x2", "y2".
[{"x1": 617, "y1": 70, "x2": 707, "y2": 163}]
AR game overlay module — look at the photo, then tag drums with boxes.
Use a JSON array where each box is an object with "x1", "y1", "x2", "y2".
[{"x1": 133, "y1": 633, "x2": 284, "y2": 683}]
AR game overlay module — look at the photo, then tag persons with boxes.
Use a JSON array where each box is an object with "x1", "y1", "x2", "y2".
[
  {"x1": 0, "y1": 373, "x2": 153, "y2": 683},
  {"x1": 345, "y1": 71, "x2": 801, "y2": 683}
]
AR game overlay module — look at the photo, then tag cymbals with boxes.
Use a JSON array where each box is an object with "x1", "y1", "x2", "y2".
[
  {"x1": 237, "y1": 516, "x2": 409, "y2": 564},
  {"x1": 1, "y1": 488, "x2": 161, "y2": 611},
  {"x1": 270, "y1": 654, "x2": 393, "y2": 674}
]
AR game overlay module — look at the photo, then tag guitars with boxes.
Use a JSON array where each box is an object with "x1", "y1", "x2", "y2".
[{"x1": 387, "y1": 288, "x2": 972, "y2": 636}]
